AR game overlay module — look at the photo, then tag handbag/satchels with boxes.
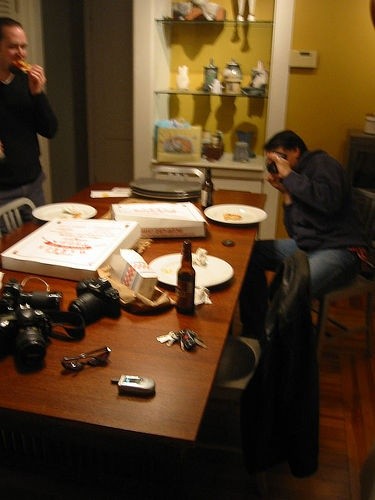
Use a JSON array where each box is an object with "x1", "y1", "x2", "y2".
[{"x1": 153, "y1": 122, "x2": 202, "y2": 162}]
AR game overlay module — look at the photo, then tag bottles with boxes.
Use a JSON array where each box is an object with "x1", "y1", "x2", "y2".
[
  {"x1": 176, "y1": 60, "x2": 268, "y2": 94},
  {"x1": 202, "y1": 130, "x2": 225, "y2": 162},
  {"x1": 201, "y1": 167, "x2": 214, "y2": 207},
  {"x1": 176, "y1": 241, "x2": 196, "y2": 315}
]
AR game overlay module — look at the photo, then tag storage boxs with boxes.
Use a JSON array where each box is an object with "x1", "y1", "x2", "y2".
[
  {"x1": 155, "y1": 125, "x2": 202, "y2": 164},
  {"x1": 111, "y1": 201, "x2": 207, "y2": 238},
  {"x1": 1, "y1": 217, "x2": 142, "y2": 282},
  {"x1": 109, "y1": 247, "x2": 158, "y2": 299}
]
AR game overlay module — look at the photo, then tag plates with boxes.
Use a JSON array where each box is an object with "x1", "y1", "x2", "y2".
[
  {"x1": 32, "y1": 203, "x2": 97, "y2": 221},
  {"x1": 148, "y1": 252, "x2": 234, "y2": 287},
  {"x1": 204, "y1": 203, "x2": 268, "y2": 224},
  {"x1": 128, "y1": 177, "x2": 201, "y2": 200}
]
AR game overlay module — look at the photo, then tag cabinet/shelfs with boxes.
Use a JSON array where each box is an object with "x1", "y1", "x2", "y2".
[{"x1": 152, "y1": 18, "x2": 274, "y2": 172}]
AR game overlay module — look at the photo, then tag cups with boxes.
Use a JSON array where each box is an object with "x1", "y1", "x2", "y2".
[
  {"x1": 363, "y1": 117, "x2": 375, "y2": 134},
  {"x1": 233, "y1": 130, "x2": 257, "y2": 162}
]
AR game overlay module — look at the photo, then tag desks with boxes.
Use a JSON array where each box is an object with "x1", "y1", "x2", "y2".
[
  {"x1": 347, "y1": 128, "x2": 375, "y2": 188},
  {"x1": 0, "y1": 182, "x2": 267, "y2": 500}
]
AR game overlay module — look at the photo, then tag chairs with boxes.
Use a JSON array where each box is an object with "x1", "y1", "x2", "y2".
[
  {"x1": 309, "y1": 187, "x2": 375, "y2": 364},
  {"x1": 163, "y1": 253, "x2": 320, "y2": 500},
  {"x1": 0, "y1": 196, "x2": 37, "y2": 238},
  {"x1": 145, "y1": 165, "x2": 204, "y2": 182}
]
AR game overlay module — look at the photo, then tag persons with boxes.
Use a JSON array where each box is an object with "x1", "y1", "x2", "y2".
[
  {"x1": 238, "y1": 129, "x2": 368, "y2": 339},
  {"x1": 0, "y1": 17, "x2": 60, "y2": 238}
]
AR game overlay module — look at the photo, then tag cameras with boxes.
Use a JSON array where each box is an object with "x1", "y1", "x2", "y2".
[
  {"x1": 267, "y1": 161, "x2": 278, "y2": 175},
  {"x1": 0, "y1": 282, "x2": 61, "y2": 371},
  {"x1": 70, "y1": 279, "x2": 123, "y2": 323}
]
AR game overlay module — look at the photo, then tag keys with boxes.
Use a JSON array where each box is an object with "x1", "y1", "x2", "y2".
[{"x1": 157, "y1": 323, "x2": 208, "y2": 351}]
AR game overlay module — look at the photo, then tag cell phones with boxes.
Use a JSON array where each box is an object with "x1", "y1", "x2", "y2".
[{"x1": 112, "y1": 374, "x2": 155, "y2": 394}]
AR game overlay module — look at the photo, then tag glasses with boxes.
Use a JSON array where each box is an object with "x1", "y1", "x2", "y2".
[{"x1": 62, "y1": 345, "x2": 112, "y2": 371}]
